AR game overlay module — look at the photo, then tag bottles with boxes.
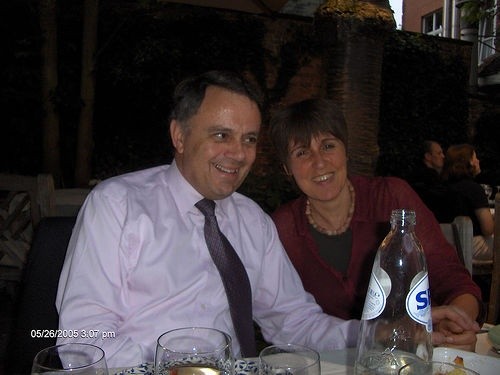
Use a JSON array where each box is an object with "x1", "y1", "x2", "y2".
[{"x1": 352, "y1": 208, "x2": 435, "y2": 374}]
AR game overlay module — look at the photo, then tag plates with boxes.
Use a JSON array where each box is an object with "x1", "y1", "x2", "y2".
[
  {"x1": 433, "y1": 347, "x2": 500, "y2": 375},
  {"x1": 115, "y1": 355, "x2": 293, "y2": 375}
]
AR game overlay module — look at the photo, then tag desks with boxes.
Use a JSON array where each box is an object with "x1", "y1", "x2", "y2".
[{"x1": 38, "y1": 323, "x2": 500, "y2": 375}]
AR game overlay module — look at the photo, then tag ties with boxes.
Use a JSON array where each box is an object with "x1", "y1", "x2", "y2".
[{"x1": 197, "y1": 197, "x2": 259, "y2": 357}]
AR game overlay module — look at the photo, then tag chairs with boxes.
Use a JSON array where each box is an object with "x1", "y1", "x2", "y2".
[
  {"x1": 438, "y1": 215, "x2": 493, "y2": 283},
  {"x1": 0, "y1": 174, "x2": 92, "y2": 374}
]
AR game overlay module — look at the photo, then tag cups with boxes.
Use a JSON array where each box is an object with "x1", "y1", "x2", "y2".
[
  {"x1": 31, "y1": 340, "x2": 111, "y2": 375},
  {"x1": 254, "y1": 344, "x2": 322, "y2": 375},
  {"x1": 154, "y1": 326, "x2": 236, "y2": 375}
]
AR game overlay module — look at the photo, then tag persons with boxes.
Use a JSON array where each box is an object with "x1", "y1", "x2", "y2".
[
  {"x1": 25, "y1": 174, "x2": 100, "y2": 344},
  {"x1": 266, "y1": 99, "x2": 484, "y2": 350},
  {"x1": 436, "y1": 145, "x2": 495, "y2": 304},
  {"x1": 406, "y1": 139, "x2": 447, "y2": 213},
  {"x1": 53, "y1": 70, "x2": 479, "y2": 375}
]
